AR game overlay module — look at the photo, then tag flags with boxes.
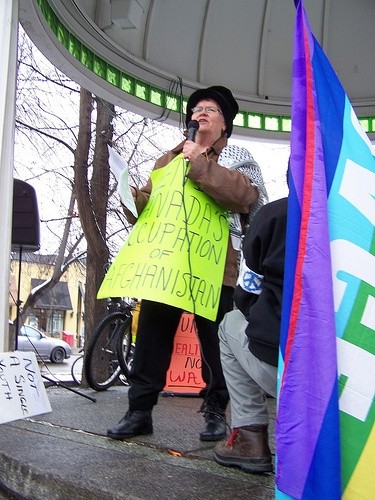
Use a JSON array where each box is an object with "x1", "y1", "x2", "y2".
[{"x1": 272, "y1": 1, "x2": 375, "y2": 500}]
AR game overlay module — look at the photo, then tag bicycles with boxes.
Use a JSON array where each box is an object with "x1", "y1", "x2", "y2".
[{"x1": 83, "y1": 295, "x2": 140, "y2": 392}]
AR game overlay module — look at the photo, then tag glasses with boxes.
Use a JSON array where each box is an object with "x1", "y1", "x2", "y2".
[{"x1": 191, "y1": 106, "x2": 223, "y2": 116}]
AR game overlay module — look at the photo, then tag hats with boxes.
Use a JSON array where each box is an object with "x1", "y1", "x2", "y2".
[{"x1": 186, "y1": 85, "x2": 240, "y2": 138}]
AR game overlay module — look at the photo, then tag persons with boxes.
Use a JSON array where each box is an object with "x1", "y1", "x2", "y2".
[
  {"x1": 212, "y1": 159, "x2": 290, "y2": 474},
  {"x1": 104, "y1": 85, "x2": 269, "y2": 443}
]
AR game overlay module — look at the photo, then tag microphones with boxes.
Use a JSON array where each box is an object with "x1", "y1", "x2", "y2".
[{"x1": 185, "y1": 120, "x2": 200, "y2": 166}]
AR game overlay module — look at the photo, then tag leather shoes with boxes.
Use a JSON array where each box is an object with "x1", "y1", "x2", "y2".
[
  {"x1": 199, "y1": 412, "x2": 227, "y2": 441},
  {"x1": 215, "y1": 423, "x2": 273, "y2": 472},
  {"x1": 107, "y1": 410, "x2": 154, "y2": 439}
]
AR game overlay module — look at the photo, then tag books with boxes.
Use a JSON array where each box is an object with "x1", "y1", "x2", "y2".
[{"x1": 104, "y1": 143, "x2": 140, "y2": 220}]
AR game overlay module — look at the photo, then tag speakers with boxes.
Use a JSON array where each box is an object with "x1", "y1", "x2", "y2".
[{"x1": 11, "y1": 179, "x2": 40, "y2": 252}]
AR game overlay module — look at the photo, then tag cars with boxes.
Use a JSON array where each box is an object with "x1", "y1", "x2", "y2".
[{"x1": 8, "y1": 321, "x2": 72, "y2": 364}]
